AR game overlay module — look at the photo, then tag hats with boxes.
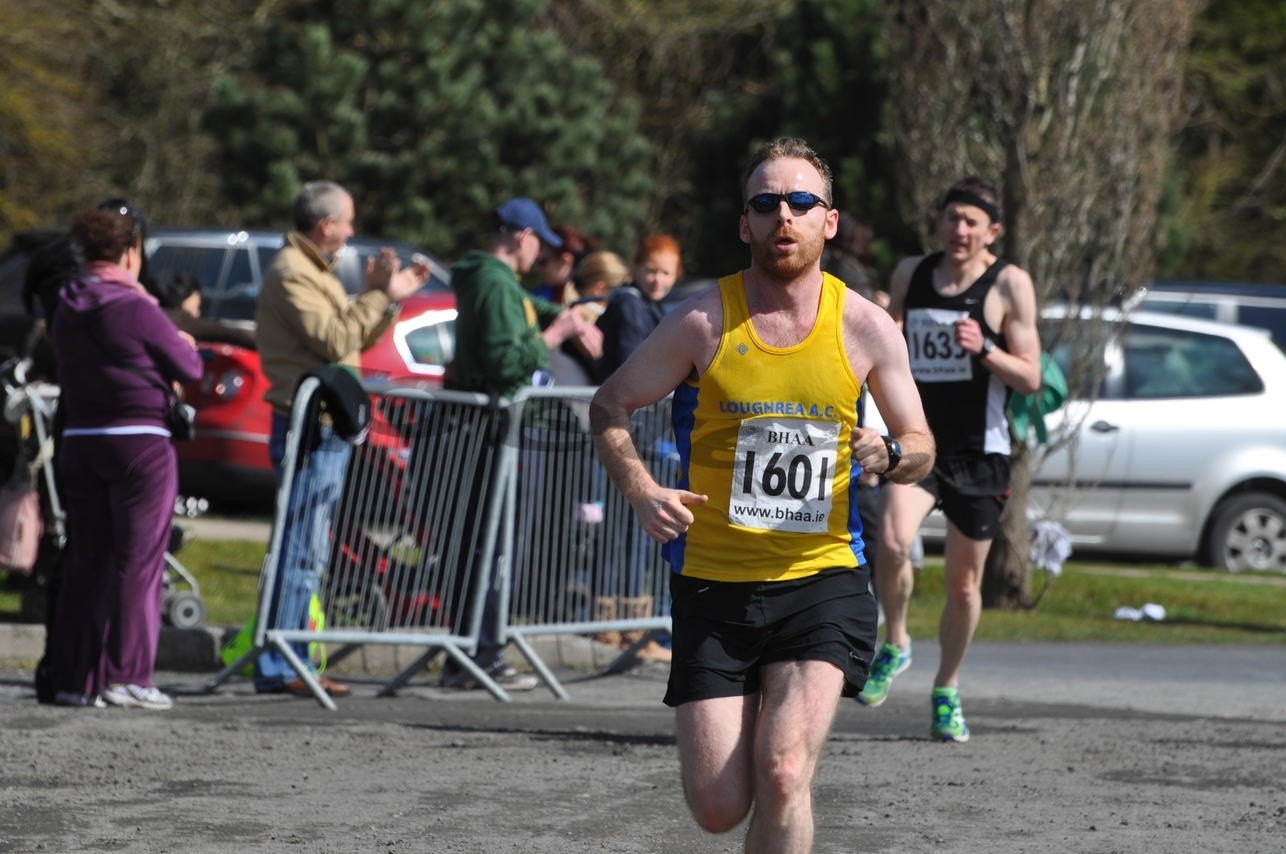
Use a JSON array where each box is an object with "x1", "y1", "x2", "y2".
[{"x1": 496, "y1": 198, "x2": 569, "y2": 249}]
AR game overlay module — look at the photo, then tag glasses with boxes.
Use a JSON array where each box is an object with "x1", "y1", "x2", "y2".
[
  {"x1": 99, "y1": 199, "x2": 141, "y2": 223},
  {"x1": 536, "y1": 259, "x2": 551, "y2": 267},
  {"x1": 742, "y1": 192, "x2": 836, "y2": 213}
]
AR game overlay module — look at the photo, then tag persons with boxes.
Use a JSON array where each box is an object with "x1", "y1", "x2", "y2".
[
  {"x1": 521, "y1": 218, "x2": 683, "y2": 667},
  {"x1": 442, "y1": 202, "x2": 602, "y2": 689},
  {"x1": 27, "y1": 201, "x2": 202, "y2": 708},
  {"x1": 253, "y1": 182, "x2": 428, "y2": 695},
  {"x1": 856, "y1": 175, "x2": 1040, "y2": 744},
  {"x1": 589, "y1": 141, "x2": 936, "y2": 854}
]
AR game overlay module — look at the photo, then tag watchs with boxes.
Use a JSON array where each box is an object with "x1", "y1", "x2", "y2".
[
  {"x1": 973, "y1": 336, "x2": 996, "y2": 360},
  {"x1": 876, "y1": 436, "x2": 902, "y2": 476}
]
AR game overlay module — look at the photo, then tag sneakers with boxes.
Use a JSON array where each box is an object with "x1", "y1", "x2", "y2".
[
  {"x1": 851, "y1": 636, "x2": 914, "y2": 708},
  {"x1": 927, "y1": 683, "x2": 977, "y2": 743}
]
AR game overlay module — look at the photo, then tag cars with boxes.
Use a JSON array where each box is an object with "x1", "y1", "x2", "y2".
[
  {"x1": 1124, "y1": 286, "x2": 1286, "y2": 355},
  {"x1": 141, "y1": 230, "x2": 458, "y2": 484},
  {"x1": 856, "y1": 307, "x2": 1286, "y2": 576}
]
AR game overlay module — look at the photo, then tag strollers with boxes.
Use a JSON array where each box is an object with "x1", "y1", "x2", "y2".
[{"x1": 8, "y1": 360, "x2": 205, "y2": 632}]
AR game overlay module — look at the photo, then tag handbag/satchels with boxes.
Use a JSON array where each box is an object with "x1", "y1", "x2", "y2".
[{"x1": 162, "y1": 392, "x2": 195, "y2": 442}]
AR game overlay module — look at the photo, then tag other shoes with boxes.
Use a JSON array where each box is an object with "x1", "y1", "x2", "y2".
[
  {"x1": 259, "y1": 675, "x2": 355, "y2": 698},
  {"x1": 102, "y1": 682, "x2": 177, "y2": 710},
  {"x1": 439, "y1": 670, "x2": 541, "y2": 691},
  {"x1": 52, "y1": 691, "x2": 95, "y2": 706}
]
